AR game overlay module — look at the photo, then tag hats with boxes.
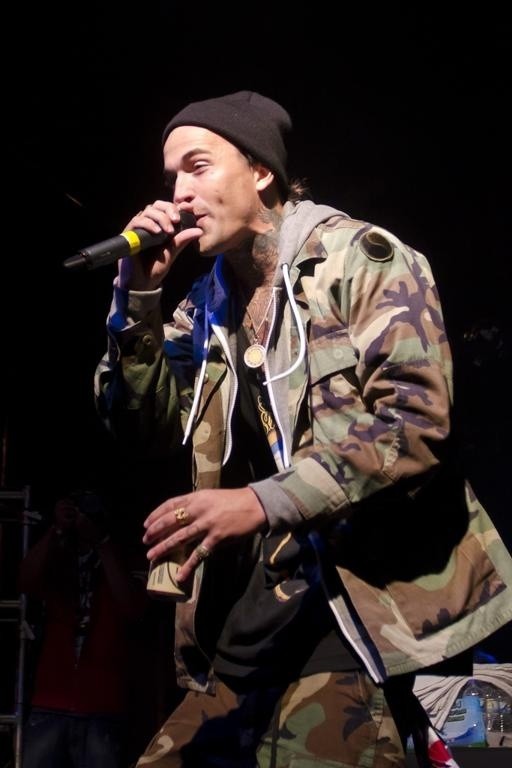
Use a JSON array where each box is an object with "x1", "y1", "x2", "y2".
[{"x1": 162, "y1": 91, "x2": 292, "y2": 197}]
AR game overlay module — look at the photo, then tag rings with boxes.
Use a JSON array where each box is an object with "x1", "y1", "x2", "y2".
[
  {"x1": 193, "y1": 544, "x2": 212, "y2": 561},
  {"x1": 171, "y1": 506, "x2": 193, "y2": 528}
]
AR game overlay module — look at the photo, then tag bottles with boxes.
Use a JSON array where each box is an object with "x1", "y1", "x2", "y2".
[{"x1": 461, "y1": 679, "x2": 512, "y2": 736}]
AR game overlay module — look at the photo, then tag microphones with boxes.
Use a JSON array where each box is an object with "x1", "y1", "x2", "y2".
[{"x1": 62, "y1": 210, "x2": 196, "y2": 274}]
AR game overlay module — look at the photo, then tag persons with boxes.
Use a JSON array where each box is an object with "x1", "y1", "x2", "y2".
[
  {"x1": 90, "y1": 90, "x2": 512, "y2": 768},
  {"x1": 5, "y1": 500, "x2": 153, "y2": 768}
]
